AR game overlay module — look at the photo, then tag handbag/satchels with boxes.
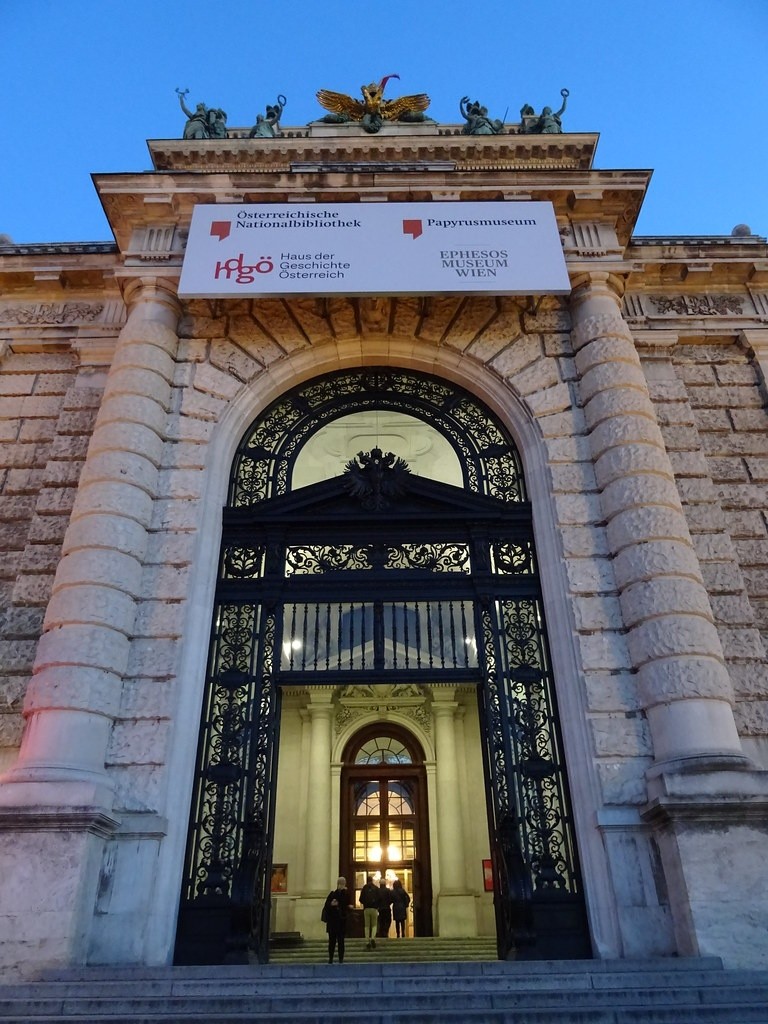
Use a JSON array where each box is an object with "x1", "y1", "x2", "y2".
[{"x1": 321, "y1": 907, "x2": 328, "y2": 922}]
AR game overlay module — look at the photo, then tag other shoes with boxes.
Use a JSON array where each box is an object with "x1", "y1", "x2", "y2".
[
  {"x1": 376, "y1": 931, "x2": 387, "y2": 937},
  {"x1": 328, "y1": 959, "x2": 333, "y2": 964},
  {"x1": 402, "y1": 933, "x2": 405, "y2": 937},
  {"x1": 397, "y1": 932, "x2": 400, "y2": 938},
  {"x1": 371, "y1": 939, "x2": 376, "y2": 948},
  {"x1": 366, "y1": 944, "x2": 371, "y2": 951},
  {"x1": 339, "y1": 957, "x2": 343, "y2": 963}
]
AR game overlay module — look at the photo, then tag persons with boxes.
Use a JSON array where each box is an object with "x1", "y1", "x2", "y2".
[
  {"x1": 180, "y1": 92, "x2": 227, "y2": 139},
  {"x1": 460, "y1": 97, "x2": 504, "y2": 133},
  {"x1": 359, "y1": 877, "x2": 411, "y2": 949},
  {"x1": 324, "y1": 877, "x2": 354, "y2": 963},
  {"x1": 520, "y1": 94, "x2": 566, "y2": 133},
  {"x1": 249, "y1": 104, "x2": 282, "y2": 138}
]
